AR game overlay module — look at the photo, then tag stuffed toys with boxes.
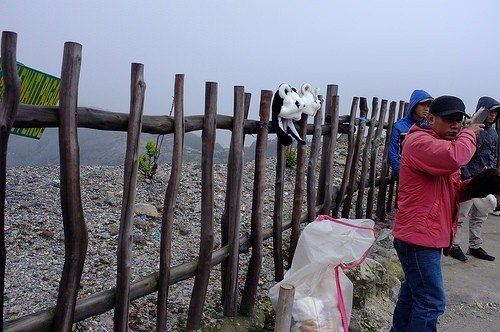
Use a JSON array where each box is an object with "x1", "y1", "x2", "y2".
[{"x1": 271, "y1": 83, "x2": 321, "y2": 145}]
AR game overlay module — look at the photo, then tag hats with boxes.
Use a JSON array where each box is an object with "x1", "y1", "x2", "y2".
[{"x1": 429, "y1": 95, "x2": 470, "y2": 119}]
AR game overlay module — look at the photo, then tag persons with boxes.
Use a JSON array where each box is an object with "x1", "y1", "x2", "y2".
[{"x1": 387, "y1": 90, "x2": 500, "y2": 331}]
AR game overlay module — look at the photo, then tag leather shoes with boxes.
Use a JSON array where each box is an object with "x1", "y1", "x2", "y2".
[
  {"x1": 449, "y1": 244, "x2": 468, "y2": 263},
  {"x1": 465, "y1": 246, "x2": 495, "y2": 261}
]
